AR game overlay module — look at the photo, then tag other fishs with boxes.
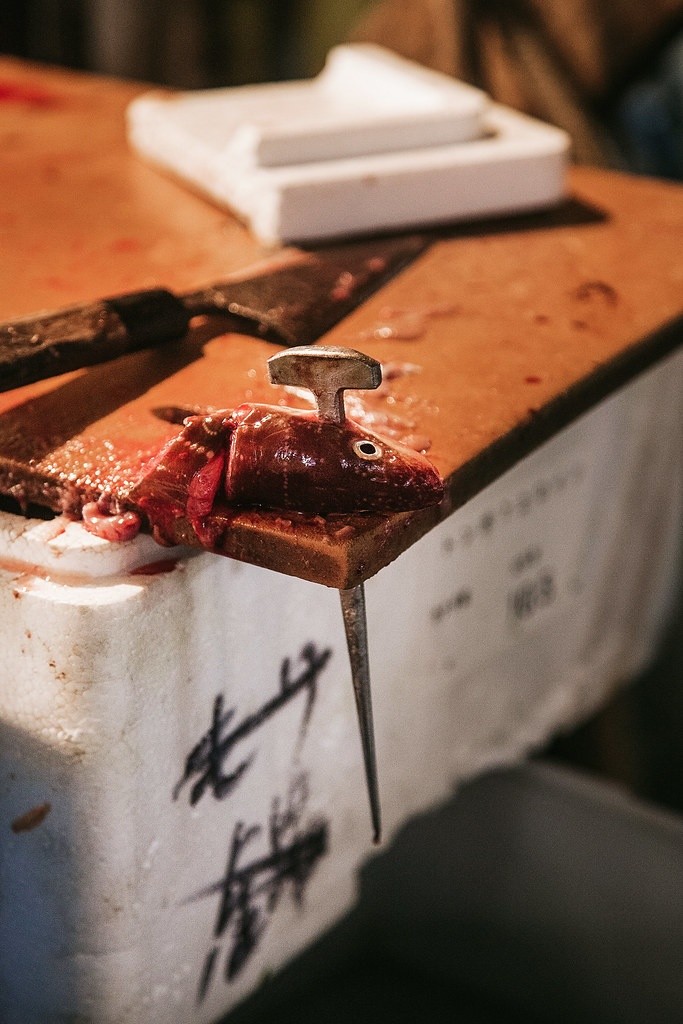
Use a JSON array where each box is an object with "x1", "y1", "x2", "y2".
[{"x1": 120, "y1": 401, "x2": 444, "y2": 548}]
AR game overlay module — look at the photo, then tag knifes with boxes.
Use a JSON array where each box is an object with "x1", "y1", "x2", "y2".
[{"x1": 0, "y1": 230, "x2": 448, "y2": 391}]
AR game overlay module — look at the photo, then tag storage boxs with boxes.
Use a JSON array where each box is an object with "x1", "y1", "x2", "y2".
[{"x1": 0, "y1": 352, "x2": 682, "y2": 1023}]
